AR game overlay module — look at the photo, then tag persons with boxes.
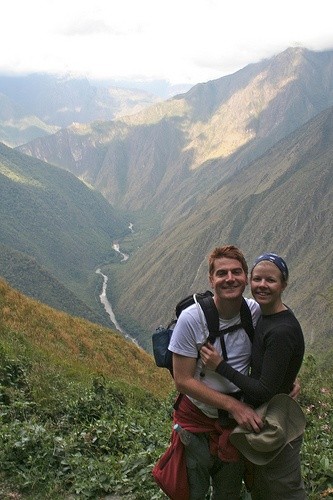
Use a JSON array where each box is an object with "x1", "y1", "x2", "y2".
[
  {"x1": 168, "y1": 244, "x2": 301, "y2": 500},
  {"x1": 201, "y1": 252, "x2": 306, "y2": 499}
]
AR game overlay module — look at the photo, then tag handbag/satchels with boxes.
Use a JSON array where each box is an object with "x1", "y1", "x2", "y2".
[
  {"x1": 153, "y1": 441, "x2": 189, "y2": 500},
  {"x1": 218, "y1": 391, "x2": 264, "y2": 428}
]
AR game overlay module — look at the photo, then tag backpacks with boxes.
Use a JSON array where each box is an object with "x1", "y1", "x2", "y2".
[{"x1": 152, "y1": 291, "x2": 254, "y2": 379}]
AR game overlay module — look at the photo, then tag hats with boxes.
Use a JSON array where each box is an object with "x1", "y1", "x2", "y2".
[
  {"x1": 230, "y1": 394, "x2": 306, "y2": 466},
  {"x1": 251, "y1": 253, "x2": 288, "y2": 281}
]
